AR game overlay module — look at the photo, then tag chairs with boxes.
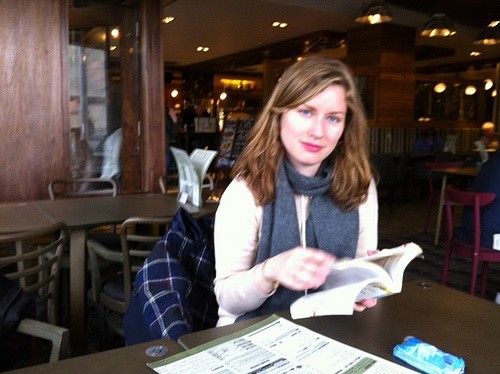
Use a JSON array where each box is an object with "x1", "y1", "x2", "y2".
[
  {"x1": 0, "y1": 177, "x2": 178, "y2": 365},
  {"x1": 441, "y1": 184, "x2": 500, "y2": 299},
  {"x1": 422, "y1": 161, "x2": 467, "y2": 234}
]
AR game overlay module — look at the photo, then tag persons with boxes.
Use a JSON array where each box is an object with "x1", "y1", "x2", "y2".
[
  {"x1": 455, "y1": 144, "x2": 500, "y2": 305},
  {"x1": 214, "y1": 56, "x2": 378, "y2": 327},
  {"x1": 180, "y1": 93, "x2": 211, "y2": 156}
]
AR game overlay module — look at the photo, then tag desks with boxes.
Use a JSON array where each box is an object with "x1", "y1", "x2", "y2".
[
  {"x1": 431, "y1": 167, "x2": 479, "y2": 245},
  {"x1": 0, "y1": 273, "x2": 500, "y2": 374},
  {"x1": 0, "y1": 192, "x2": 199, "y2": 357}
]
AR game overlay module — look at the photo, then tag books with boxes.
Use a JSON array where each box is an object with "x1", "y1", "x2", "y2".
[{"x1": 290, "y1": 243, "x2": 422, "y2": 319}]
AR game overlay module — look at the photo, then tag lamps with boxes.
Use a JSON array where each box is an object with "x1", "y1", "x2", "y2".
[{"x1": 353, "y1": 0, "x2": 500, "y2": 46}]
